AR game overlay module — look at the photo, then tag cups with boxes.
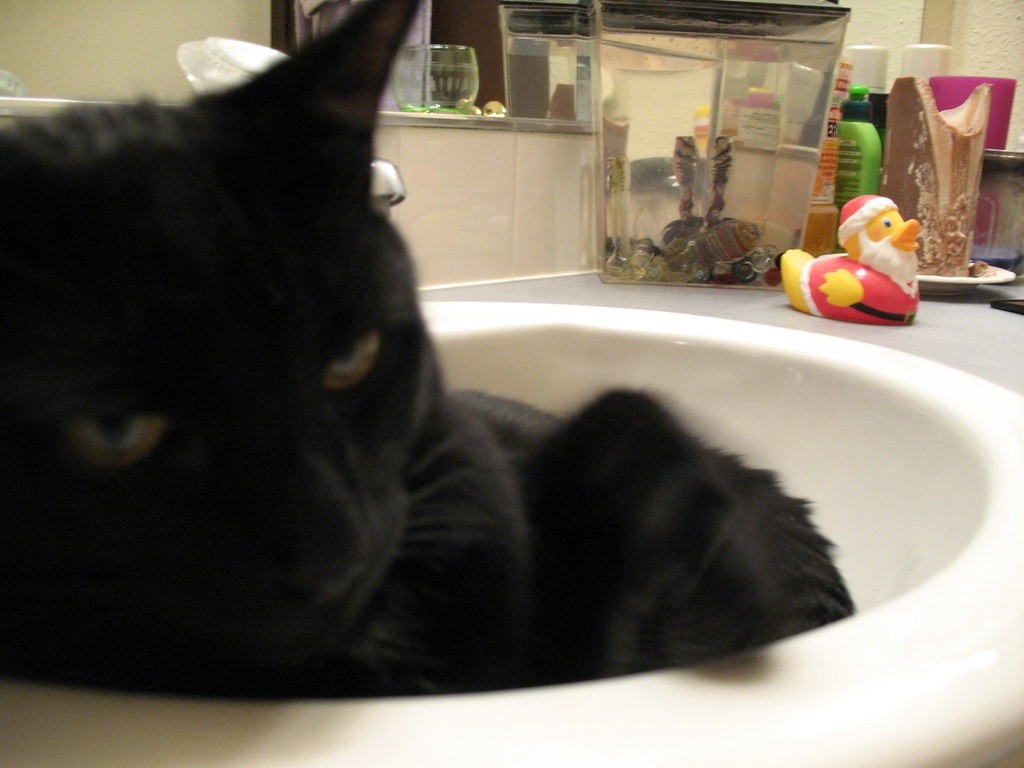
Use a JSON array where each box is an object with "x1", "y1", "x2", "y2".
[
  {"x1": 927, "y1": 75, "x2": 1016, "y2": 252},
  {"x1": 390, "y1": 43, "x2": 479, "y2": 114}
]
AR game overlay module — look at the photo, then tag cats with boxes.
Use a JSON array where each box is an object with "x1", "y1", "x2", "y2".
[{"x1": 0, "y1": 0, "x2": 857, "y2": 702}]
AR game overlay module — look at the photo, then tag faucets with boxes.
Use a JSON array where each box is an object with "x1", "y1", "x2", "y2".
[{"x1": 366, "y1": 159, "x2": 407, "y2": 208}]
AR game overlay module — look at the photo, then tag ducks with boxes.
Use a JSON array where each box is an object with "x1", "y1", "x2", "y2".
[{"x1": 778, "y1": 195, "x2": 921, "y2": 325}]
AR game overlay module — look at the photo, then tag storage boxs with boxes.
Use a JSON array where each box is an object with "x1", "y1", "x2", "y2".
[{"x1": 498, "y1": 1, "x2": 852, "y2": 293}]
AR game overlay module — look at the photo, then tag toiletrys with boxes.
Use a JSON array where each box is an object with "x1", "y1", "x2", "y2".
[{"x1": 837, "y1": 82, "x2": 883, "y2": 209}]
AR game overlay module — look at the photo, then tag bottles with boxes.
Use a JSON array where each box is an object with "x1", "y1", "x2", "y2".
[
  {"x1": 900, "y1": 42, "x2": 953, "y2": 86},
  {"x1": 845, "y1": 44, "x2": 890, "y2": 94}
]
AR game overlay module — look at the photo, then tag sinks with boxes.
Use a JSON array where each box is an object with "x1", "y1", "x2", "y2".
[{"x1": 0, "y1": 302, "x2": 1024, "y2": 768}]
